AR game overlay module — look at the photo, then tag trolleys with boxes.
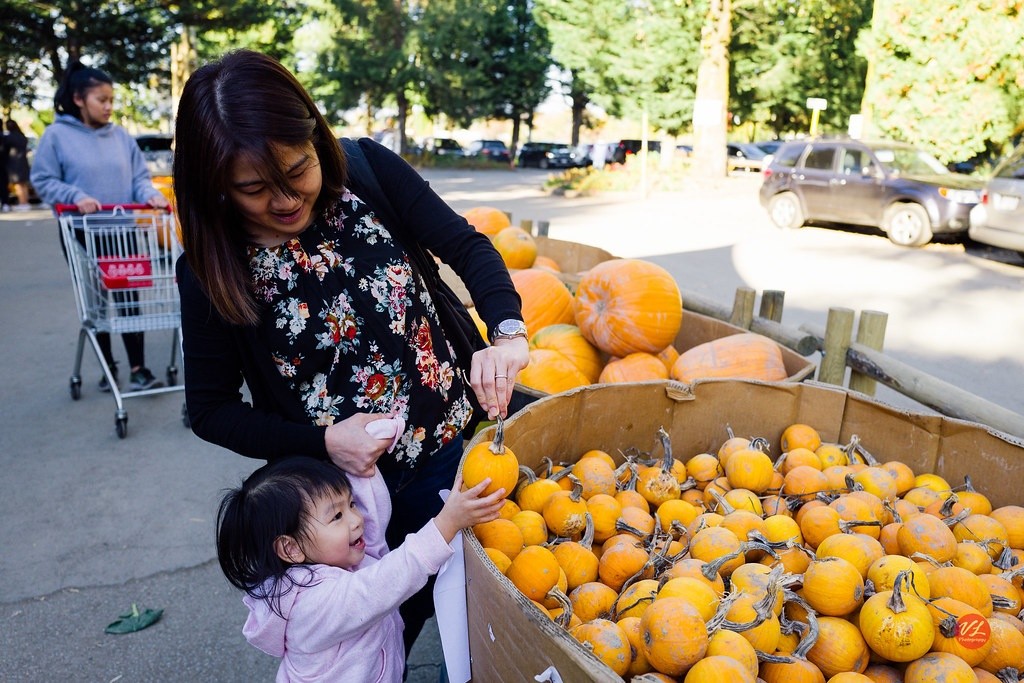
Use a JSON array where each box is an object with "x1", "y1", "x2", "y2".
[{"x1": 55, "y1": 203, "x2": 191, "y2": 438}]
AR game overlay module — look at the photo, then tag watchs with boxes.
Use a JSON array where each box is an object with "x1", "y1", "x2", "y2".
[{"x1": 491, "y1": 319, "x2": 529, "y2": 345}]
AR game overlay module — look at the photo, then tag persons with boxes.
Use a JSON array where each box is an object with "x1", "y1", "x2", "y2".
[
  {"x1": 172, "y1": 48, "x2": 532, "y2": 683},
  {"x1": 0, "y1": 118, "x2": 30, "y2": 213},
  {"x1": 30, "y1": 60, "x2": 173, "y2": 395},
  {"x1": 613, "y1": 142, "x2": 627, "y2": 165},
  {"x1": 214, "y1": 455, "x2": 508, "y2": 683}
]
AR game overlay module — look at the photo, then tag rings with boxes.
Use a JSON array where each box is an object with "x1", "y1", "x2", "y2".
[{"x1": 494, "y1": 375, "x2": 508, "y2": 379}]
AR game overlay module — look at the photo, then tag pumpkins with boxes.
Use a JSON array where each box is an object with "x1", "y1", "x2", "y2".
[
  {"x1": 131, "y1": 174, "x2": 184, "y2": 249},
  {"x1": 461, "y1": 418, "x2": 1024, "y2": 683},
  {"x1": 430, "y1": 206, "x2": 788, "y2": 396}
]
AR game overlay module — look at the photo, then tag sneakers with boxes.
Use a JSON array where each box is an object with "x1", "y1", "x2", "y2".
[
  {"x1": 98, "y1": 360, "x2": 121, "y2": 391},
  {"x1": 130, "y1": 367, "x2": 164, "y2": 390}
]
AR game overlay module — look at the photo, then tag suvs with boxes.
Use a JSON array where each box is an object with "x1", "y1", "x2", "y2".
[
  {"x1": 757, "y1": 137, "x2": 981, "y2": 246},
  {"x1": 519, "y1": 143, "x2": 576, "y2": 169}
]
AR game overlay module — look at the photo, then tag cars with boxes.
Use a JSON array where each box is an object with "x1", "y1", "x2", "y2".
[
  {"x1": 605, "y1": 139, "x2": 783, "y2": 173},
  {"x1": 133, "y1": 135, "x2": 174, "y2": 175},
  {"x1": 472, "y1": 140, "x2": 510, "y2": 163},
  {"x1": 431, "y1": 139, "x2": 464, "y2": 157},
  {"x1": 968, "y1": 143, "x2": 1024, "y2": 252}
]
